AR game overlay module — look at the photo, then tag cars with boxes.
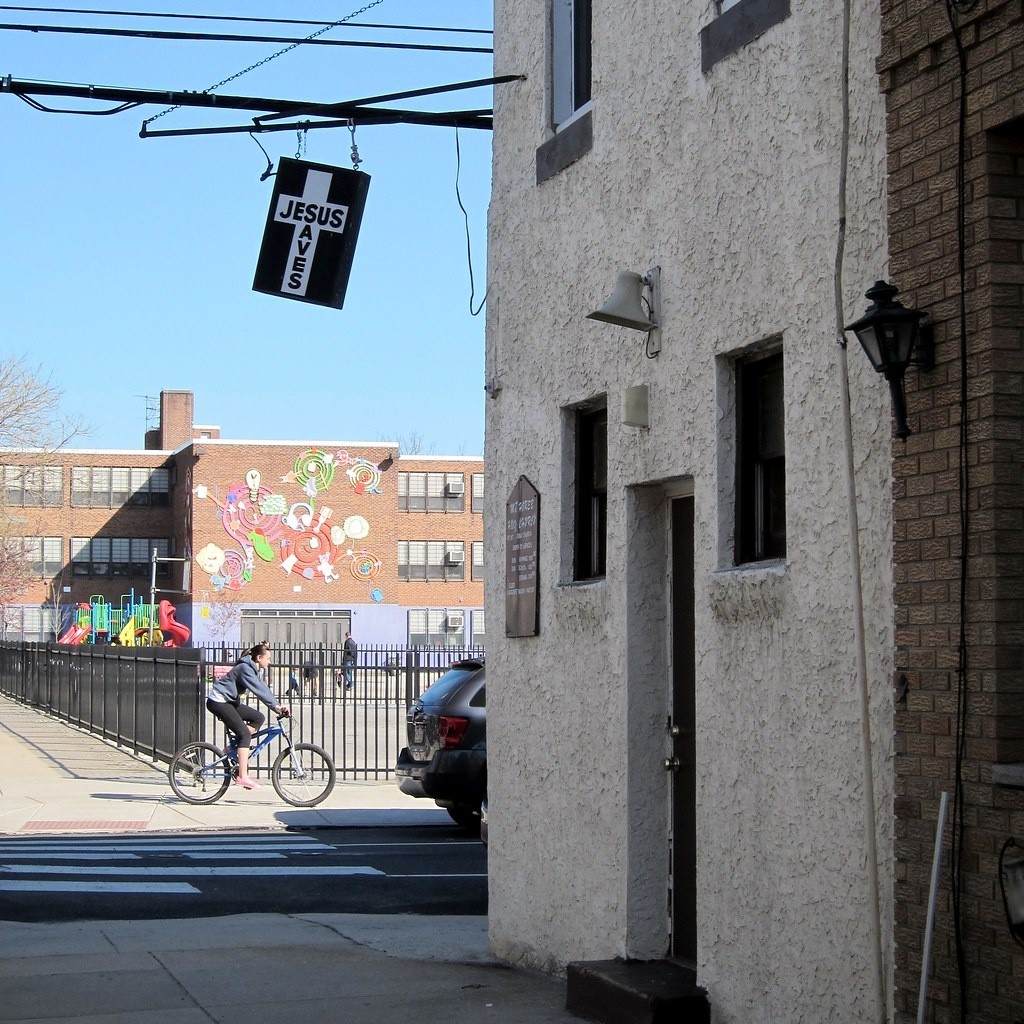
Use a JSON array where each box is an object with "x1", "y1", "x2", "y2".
[{"x1": 394, "y1": 656, "x2": 487, "y2": 824}]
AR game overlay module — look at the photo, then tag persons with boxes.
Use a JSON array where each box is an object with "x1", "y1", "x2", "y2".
[
  {"x1": 207, "y1": 645, "x2": 290, "y2": 790},
  {"x1": 263, "y1": 640, "x2": 269, "y2": 688},
  {"x1": 342, "y1": 632, "x2": 357, "y2": 690},
  {"x1": 334, "y1": 669, "x2": 342, "y2": 688},
  {"x1": 285, "y1": 666, "x2": 301, "y2": 696},
  {"x1": 300, "y1": 661, "x2": 317, "y2": 696}
]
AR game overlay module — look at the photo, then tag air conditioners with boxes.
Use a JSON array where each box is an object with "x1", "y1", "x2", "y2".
[
  {"x1": 445, "y1": 551, "x2": 464, "y2": 564},
  {"x1": 447, "y1": 615, "x2": 463, "y2": 626},
  {"x1": 446, "y1": 481, "x2": 464, "y2": 495}
]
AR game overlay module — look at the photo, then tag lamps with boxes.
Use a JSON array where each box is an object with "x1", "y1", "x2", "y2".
[
  {"x1": 586, "y1": 266, "x2": 663, "y2": 359},
  {"x1": 843, "y1": 280, "x2": 935, "y2": 444}
]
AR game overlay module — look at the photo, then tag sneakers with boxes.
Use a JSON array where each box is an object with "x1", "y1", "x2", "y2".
[
  {"x1": 234, "y1": 776, "x2": 259, "y2": 789},
  {"x1": 222, "y1": 747, "x2": 240, "y2": 763}
]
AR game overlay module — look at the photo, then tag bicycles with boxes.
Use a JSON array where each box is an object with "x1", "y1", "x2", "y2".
[{"x1": 169, "y1": 711, "x2": 336, "y2": 807}]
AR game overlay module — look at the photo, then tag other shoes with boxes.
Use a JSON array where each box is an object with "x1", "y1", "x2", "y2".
[{"x1": 345, "y1": 685, "x2": 353, "y2": 691}]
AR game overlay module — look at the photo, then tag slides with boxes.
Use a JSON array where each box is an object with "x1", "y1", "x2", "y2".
[
  {"x1": 162, "y1": 621, "x2": 191, "y2": 648},
  {"x1": 59, "y1": 625, "x2": 90, "y2": 645}
]
